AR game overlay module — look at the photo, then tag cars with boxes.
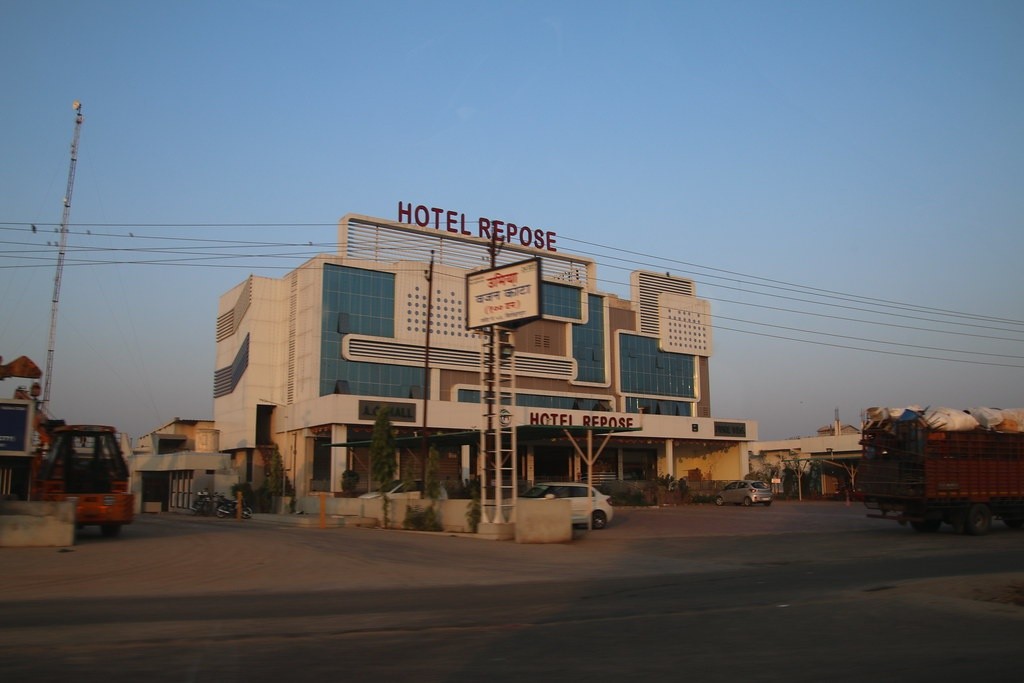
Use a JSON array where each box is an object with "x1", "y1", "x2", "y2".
[{"x1": 713, "y1": 480, "x2": 774, "y2": 506}]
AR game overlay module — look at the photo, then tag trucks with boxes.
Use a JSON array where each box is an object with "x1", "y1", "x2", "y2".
[{"x1": 853, "y1": 416, "x2": 1024, "y2": 535}]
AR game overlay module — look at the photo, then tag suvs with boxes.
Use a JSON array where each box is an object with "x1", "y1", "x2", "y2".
[{"x1": 501, "y1": 481, "x2": 614, "y2": 529}]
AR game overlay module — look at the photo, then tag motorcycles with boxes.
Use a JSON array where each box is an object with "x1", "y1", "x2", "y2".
[{"x1": 191, "y1": 490, "x2": 253, "y2": 519}]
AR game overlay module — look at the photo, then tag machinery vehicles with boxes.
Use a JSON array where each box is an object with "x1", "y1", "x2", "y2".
[{"x1": 0, "y1": 352, "x2": 134, "y2": 537}]
